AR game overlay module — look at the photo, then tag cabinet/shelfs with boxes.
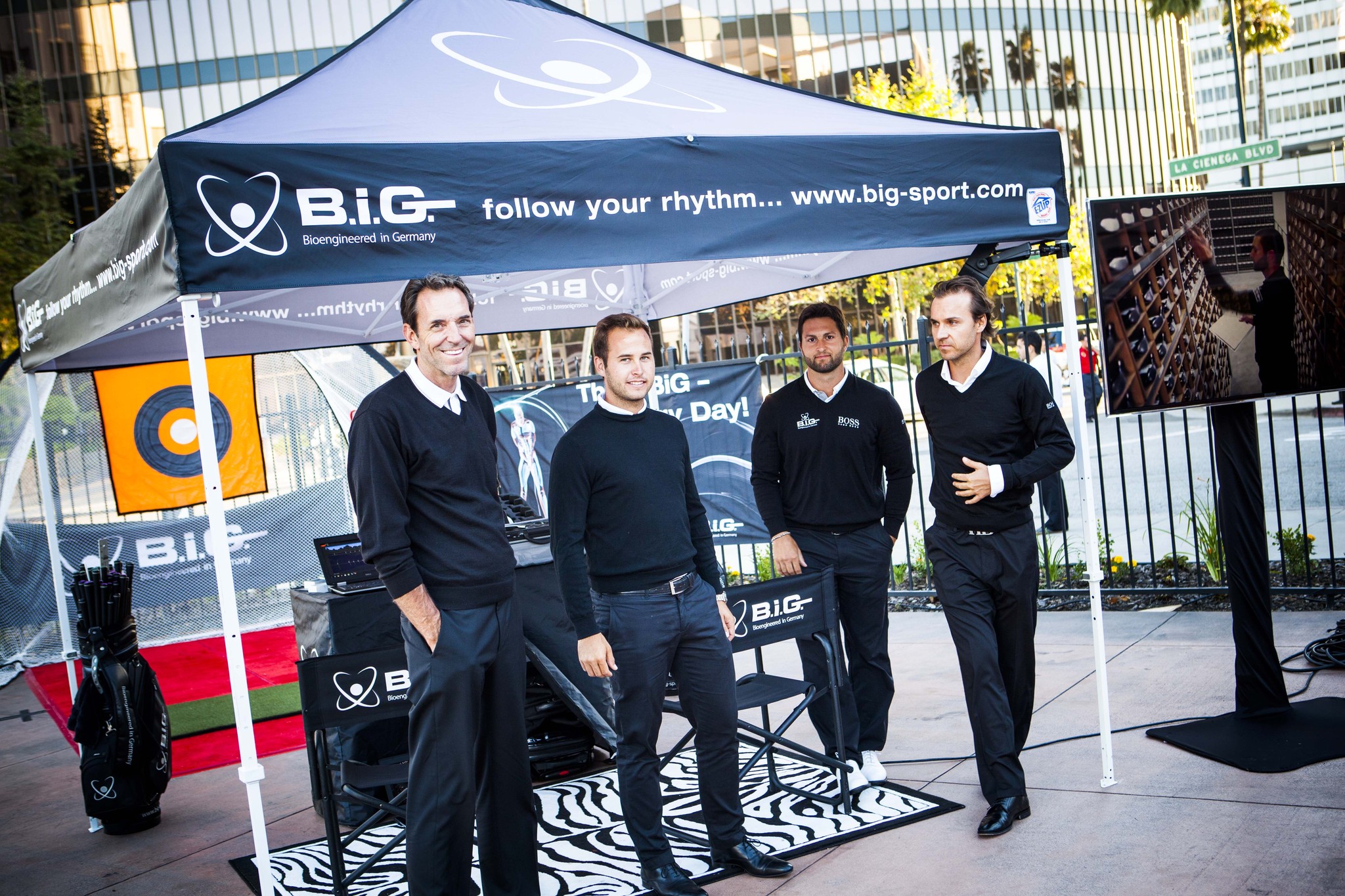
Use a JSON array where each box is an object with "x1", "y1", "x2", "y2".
[{"x1": 1093, "y1": 186, "x2": 1345, "y2": 409}]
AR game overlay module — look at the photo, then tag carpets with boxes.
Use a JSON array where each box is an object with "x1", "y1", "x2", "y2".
[
  {"x1": 228, "y1": 741, "x2": 965, "y2": 896},
  {"x1": 22, "y1": 623, "x2": 308, "y2": 776}
]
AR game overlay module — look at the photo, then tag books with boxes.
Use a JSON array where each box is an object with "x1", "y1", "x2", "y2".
[{"x1": 1209, "y1": 310, "x2": 1254, "y2": 352}]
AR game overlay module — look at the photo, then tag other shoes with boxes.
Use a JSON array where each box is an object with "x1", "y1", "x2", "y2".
[
  {"x1": 1035, "y1": 524, "x2": 1063, "y2": 534},
  {"x1": 1087, "y1": 417, "x2": 1093, "y2": 422}
]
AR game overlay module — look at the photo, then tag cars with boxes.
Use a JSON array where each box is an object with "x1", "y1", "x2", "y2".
[
  {"x1": 1035, "y1": 322, "x2": 1099, "y2": 351},
  {"x1": 844, "y1": 359, "x2": 920, "y2": 394}
]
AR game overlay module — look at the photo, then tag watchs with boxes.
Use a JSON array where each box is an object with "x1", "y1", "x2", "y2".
[{"x1": 716, "y1": 591, "x2": 727, "y2": 603}]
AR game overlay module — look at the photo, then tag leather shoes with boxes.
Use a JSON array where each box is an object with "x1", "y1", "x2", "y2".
[
  {"x1": 977, "y1": 796, "x2": 1031, "y2": 836},
  {"x1": 835, "y1": 752, "x2": 871, "y2": 795},
  {"x1": 710, "y1": 836, "x2": 793, "y2": 877},
  {"x1": 641, "y1": 862, "x2": 709, "y2": 896},
  {"x1": 860, "y1": 750, "x2": 887, "y2": 784}
]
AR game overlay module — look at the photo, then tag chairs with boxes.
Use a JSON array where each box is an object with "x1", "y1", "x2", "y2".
[
  {"x1": 660, "y1": 563, "x2": 854, "y2": 848},
  {"x1": 295, "y1": 642, "x2": 479, "y2": 896}
]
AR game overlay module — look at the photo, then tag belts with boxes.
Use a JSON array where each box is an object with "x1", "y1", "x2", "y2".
[
  {"x1": 968, "y1": 530, "x2": 994, "y2": 536},
  {"x1": 619, "y1": 570, "x2": 698, "y2": 596}
]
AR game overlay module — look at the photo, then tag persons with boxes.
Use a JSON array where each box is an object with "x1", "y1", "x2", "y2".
[
  {"x1": 915, "y1": 275, "x2": 1075, "y2": 836},
  {"x1": 750, "y1": 301, "x2": 914, "y2": 794},
  {"x1": 1187, "y1": 225, "x2": 1297, "y2": 393},
  {"x1": 548, "y1": 313, "x2": 795, "y2": 896},
  {"x1": 1039, "y1": 333, "x2": 1068, "y2": 370},
  {"x1": 1016, "y1": 331, "x2": 1069, "y2": 535},
  {"x1": 348, "y1": 274, "x2": 540, "y2": 896},
  {"x1": 1080, "y1": 334, "x2": 1103, "y2": 422}
]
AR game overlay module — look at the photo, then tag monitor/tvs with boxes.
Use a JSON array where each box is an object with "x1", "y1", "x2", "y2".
[{"x1": 1085, "y1": 182, "x2": 1345, "y2": 418}]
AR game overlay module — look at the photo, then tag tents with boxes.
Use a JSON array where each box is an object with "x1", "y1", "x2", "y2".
[
  {"x1": 842, "y1": 356, "x2": 924, "y2": 420},
  {"x1": 0, "y1": 343, "x2": 400, "y2": 671},
  {"x1": 11, "y1": 0, "x2": 1122, "y2": 896}
]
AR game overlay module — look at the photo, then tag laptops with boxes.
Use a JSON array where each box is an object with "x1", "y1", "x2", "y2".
[{"x1": 313, "y1": 532, "x2": 387, "y2": 596}]
